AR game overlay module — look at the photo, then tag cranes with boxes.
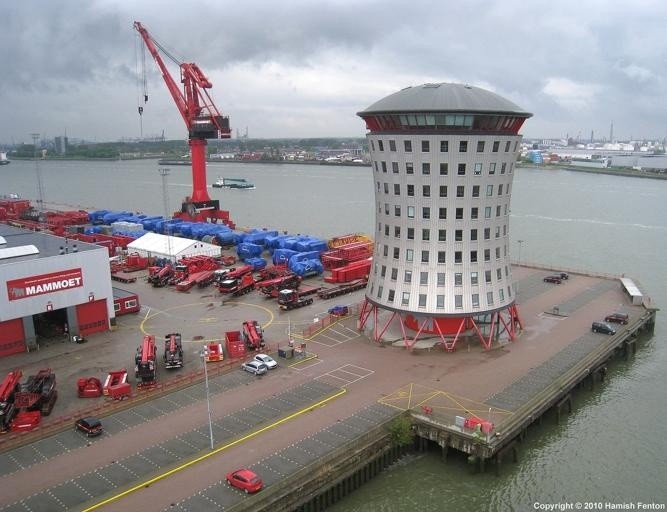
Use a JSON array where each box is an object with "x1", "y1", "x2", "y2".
[{"x1": 133, "y1": 20, "x2": 237, "y2": 229}]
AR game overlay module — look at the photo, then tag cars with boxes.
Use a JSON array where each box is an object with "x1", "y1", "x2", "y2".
[
  {"x1": 241, "y1": 354, "x2": 278, "y2": 376},
  {"x1": 544, "y1": 272, "x2": 569, "y2": 284},
  {"x1": 328, "y1": 305, "x2": 348, "y2": 316},
  {"x1": 592, "y1": 313, "x2": 629, "y2": 334},
  {"x1": 75, "y1": 416, "x2": 103, "y2": 437},
  {"x1": 226, "y1": 469, "x2": 263, "y2": 494}
]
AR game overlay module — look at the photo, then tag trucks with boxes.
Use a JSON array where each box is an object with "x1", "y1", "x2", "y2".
[
  {"x1": 279, "y1": 289, "x2": 312, "y2": 310},
  {"x1": 243, "y1": 320, "x2": 265, "y2": 351},
  {"x1": 152, "y1": 265, "x2": 254, "y2": 296},
  {"x1": 134, "y1": 333, "x2": 184, "y2": 386}
]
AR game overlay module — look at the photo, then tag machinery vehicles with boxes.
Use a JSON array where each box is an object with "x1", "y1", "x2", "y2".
[{"x1": 0, "y1": 367, "x2": 57, "y2": 431}]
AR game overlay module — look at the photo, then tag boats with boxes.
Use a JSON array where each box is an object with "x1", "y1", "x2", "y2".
[
  {"x1": 158, "y1": 159, "x2": 192, "y2": 165},
  {"x1": 0, "y1": 151, "x2": 11, "y2": 166},
  {"x1": 212, "y1": 177, "x2": 254, "y2": 189}
]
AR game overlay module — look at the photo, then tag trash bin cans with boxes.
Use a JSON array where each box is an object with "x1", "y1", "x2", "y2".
[
  {"x1": 553, "y1": 305, "x2": 560, "y2": 316},
  {"x1": 110, "y1": 317, "x2": 116, "y2": 326}
]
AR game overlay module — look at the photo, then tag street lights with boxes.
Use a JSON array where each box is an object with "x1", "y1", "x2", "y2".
[
  {"x1": 199, "y1": 344, "x2": 213, "y2": 449},
  {"x1": 517, "y1": 240, "x2": 524, "y2": 266}
]
